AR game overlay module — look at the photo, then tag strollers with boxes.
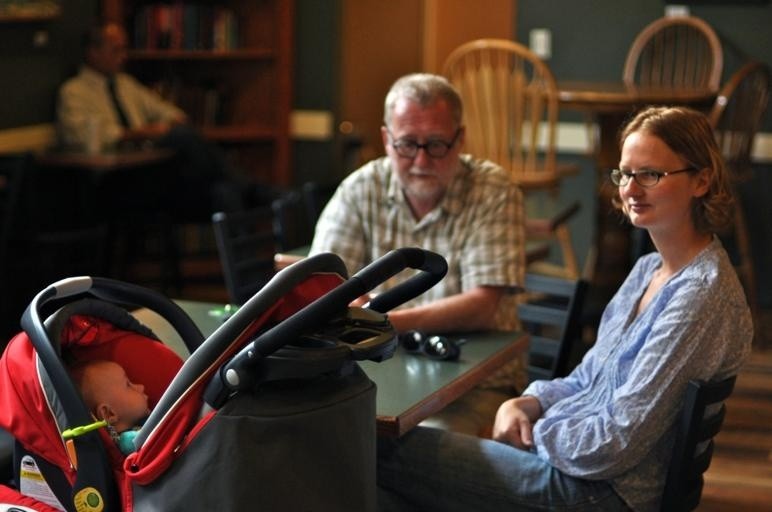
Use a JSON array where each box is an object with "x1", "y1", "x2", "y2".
[{"x1": 0, "y1": 247, "x2": 450, "y2": 512}]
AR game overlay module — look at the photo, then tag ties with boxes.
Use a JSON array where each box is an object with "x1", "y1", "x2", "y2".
[{"x1": 108, "y1": 79, "x2": 130, "y2": 129}]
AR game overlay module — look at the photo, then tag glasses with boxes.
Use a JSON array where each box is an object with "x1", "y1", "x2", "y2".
[
  {"x1": 386, "y1": 125, "x2": 462, "y2": 158},
  {"x1": 399, "y1": 329, "x2": 467, "y2": 359},
  {"x1": 609, "y1": 168, "x2": 695, "y2": 187}
]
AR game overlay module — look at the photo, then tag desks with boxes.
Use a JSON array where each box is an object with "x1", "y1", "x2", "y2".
[
  {"x1": 126, "y1": 298, "x2": 529, "y2": 437},
  {"x1": 524, "y1": 79, "x2": 716, "y2": 279}
]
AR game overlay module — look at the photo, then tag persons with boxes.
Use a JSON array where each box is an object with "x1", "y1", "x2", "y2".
[
  {"x1": 381, "y1": 103, "x2": 756, "y2": 509},
  {"x1": 56, "y1": 18, "x2": 191, "y2": 157},
  {"x1": 75, "y1": 353, "x2": 151, "y2": 456},
  {"x1": 308, "y1": 67, "x2": 529, "y2": 396}
]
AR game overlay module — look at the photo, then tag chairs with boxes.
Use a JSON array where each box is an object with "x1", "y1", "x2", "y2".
[
  {"x1": 621, "y1": 15, "x2": 723, "y2": 89},
  {"x1": 517, "y1": 275, "x2": 589, "y2": 391},
  {"x1": 440, "y1": 38, "x2": 581, "y2": 278},
  {"x1": 598, "y1": 60, "x2": 771, "y2": 350},
  {"x1": 661, "y1": 376, "x2": 737, "y2": 512}
]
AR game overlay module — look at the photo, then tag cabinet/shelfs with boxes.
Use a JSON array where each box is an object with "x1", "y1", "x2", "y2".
[{"x1": 91, "y1": 1, "x2": 294, "y2": 277}]
